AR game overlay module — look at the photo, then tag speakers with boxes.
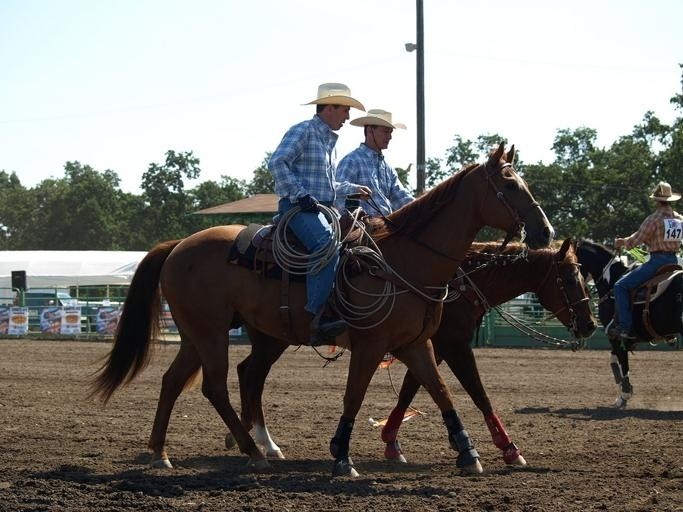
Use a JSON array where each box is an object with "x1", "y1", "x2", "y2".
[{"x1": 12, "y1": 271, "x2": 26, "y2": 291}]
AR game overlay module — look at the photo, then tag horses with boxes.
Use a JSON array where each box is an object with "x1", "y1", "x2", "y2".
[
  {"x1": 84, "y1": 140, "x2": 554, "y2": 475},
  {"x1": 573, "y1": 237, "x2": 683, "y2": 411},
  {"x1": 224, "y1": 235, "x2": 598, "y2": 467}
]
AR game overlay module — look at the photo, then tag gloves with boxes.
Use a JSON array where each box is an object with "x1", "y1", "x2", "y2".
[{"x1": 297, "y1": 194, "x2": 320, "y2": 213}]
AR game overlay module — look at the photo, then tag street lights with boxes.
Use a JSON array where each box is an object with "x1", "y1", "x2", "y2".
[{"x1": 402, "y1": 40, "x2": 425, "y2": 198}]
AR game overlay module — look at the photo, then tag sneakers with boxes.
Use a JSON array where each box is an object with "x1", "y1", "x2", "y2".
[
  {"x1": 305, "y1": 313, "x2": 348, "y2": 344},
  {"x1": 608, "y1": 326, "x2": 631, "y2": 339}
]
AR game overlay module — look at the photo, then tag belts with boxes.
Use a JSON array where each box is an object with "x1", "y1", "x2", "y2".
[{"x1": 650, "y1": 251, "x2": 677, "y2": 256}]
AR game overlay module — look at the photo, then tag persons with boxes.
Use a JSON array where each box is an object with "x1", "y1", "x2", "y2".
[
  {"x1": 611, "y1": 180, "x2": 683, "y2": 338},
  {"x1": 340, "y1": 109, "x2": 416, "y2": 219},
  {"x1": 269, "y1": 83, "x2": 372, "y2": 340}
]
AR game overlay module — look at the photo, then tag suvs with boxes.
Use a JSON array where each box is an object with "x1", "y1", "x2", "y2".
[{"x1": 12, "y1": 291, "x2": 102, "y2": 331}]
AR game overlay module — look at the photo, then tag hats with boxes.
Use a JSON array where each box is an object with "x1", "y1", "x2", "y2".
[
  {"x1": 300, "y1": 82, "x2": 366, "y2": 113},
  {"x1": 350, "y1": 109, "x2": 408, "y2": 131},
  {"x1": 648, "y1": 180, "x2": 682, "y2": 203}
]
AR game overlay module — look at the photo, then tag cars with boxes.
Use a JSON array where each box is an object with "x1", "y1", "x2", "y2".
[
  {"x1": 502, "y1": 292, "x2": 541, "y2": 320},
  {"x1": 98, "y1": 302, "x2": 176, "y2": 333}
]
